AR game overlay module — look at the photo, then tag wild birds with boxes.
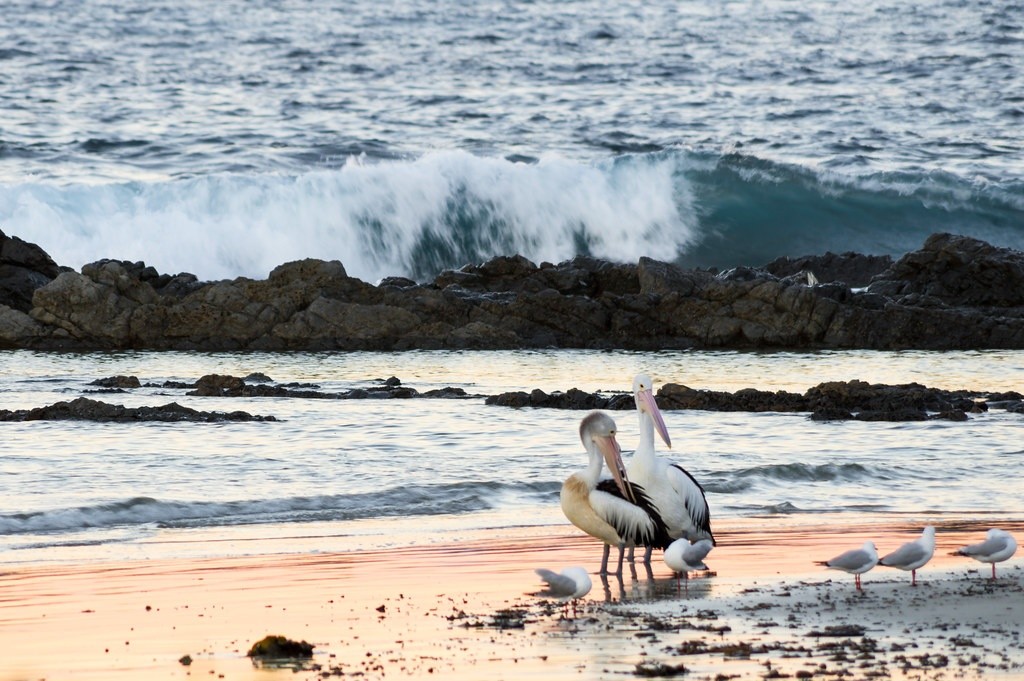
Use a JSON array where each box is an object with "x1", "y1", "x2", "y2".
[
  {"x1": 559, "y1": 373, "x2": 716, "y2": 581},
  {"x1": 664, "y1": 537, "x2": 713, "y2": 600},
  {"x1": 812, "y1": 540, "x2": 878, "y2": 590},
  {"x1": 874, "y1": 526, "x2": 935, "y2": 586},
  {"x1": 947, "y1": 528, "x2": 1017, "y2": 581},
  {"x1": 523, "y1": 566, "x2": 591, "y2": 621}
]
